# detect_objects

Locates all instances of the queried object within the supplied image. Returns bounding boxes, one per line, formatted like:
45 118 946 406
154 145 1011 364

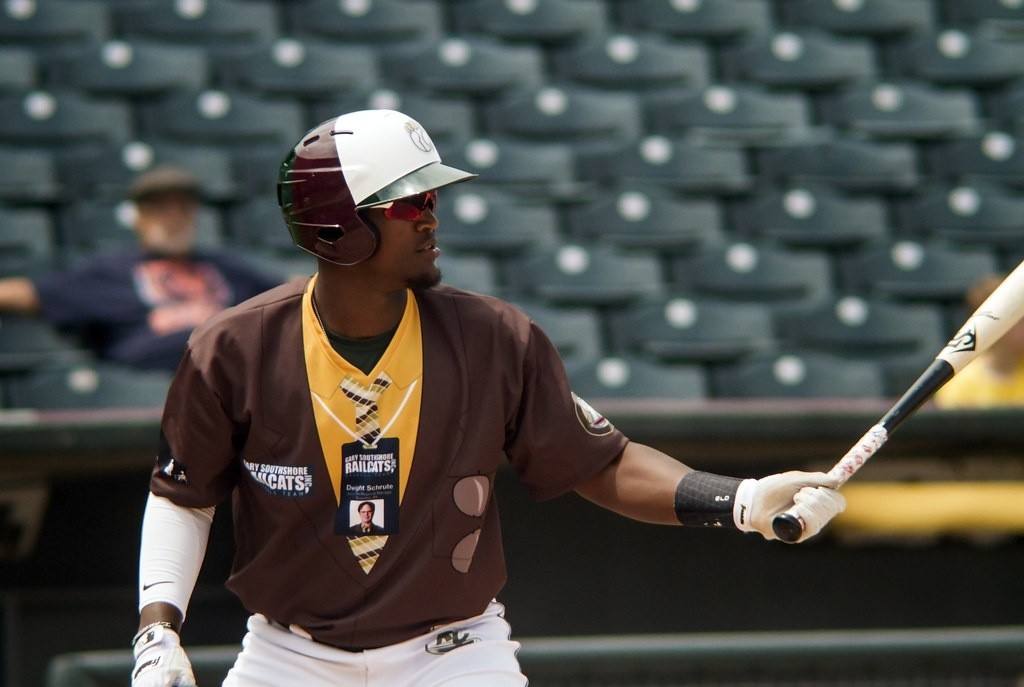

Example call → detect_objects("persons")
935 275 1024 410
131 108 847 687
0 165 285 376
351 502 382 536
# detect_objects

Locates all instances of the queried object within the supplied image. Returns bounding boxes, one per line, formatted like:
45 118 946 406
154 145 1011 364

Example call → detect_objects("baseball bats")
772 256 1024 545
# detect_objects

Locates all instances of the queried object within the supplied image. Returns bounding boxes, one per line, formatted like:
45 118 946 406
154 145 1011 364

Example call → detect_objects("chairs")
0 0 1024 409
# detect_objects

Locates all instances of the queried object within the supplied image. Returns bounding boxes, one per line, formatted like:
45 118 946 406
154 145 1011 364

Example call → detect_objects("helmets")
276 106 479 270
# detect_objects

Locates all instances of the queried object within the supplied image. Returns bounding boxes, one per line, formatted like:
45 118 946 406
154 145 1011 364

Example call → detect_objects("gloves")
130 626 195 687
733 469 848 545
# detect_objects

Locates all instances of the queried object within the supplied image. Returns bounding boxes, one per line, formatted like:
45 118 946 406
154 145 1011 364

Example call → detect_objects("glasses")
358 186 438 224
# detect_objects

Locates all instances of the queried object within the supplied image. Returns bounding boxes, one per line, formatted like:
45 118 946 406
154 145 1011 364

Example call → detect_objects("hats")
130 165 205 202
